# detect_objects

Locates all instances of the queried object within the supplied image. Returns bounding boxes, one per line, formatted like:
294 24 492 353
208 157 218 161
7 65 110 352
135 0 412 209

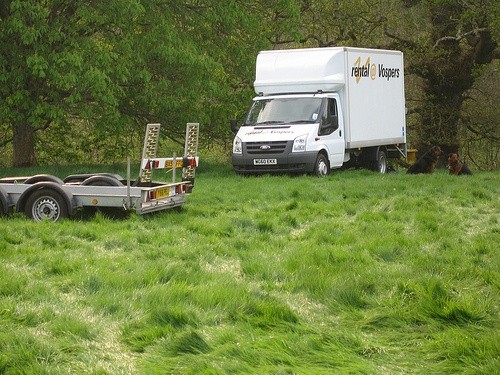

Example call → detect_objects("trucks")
230 47 407 177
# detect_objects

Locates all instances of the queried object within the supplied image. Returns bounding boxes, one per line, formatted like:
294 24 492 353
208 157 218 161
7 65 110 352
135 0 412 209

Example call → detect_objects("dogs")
405 146 444 174
446 153 472 175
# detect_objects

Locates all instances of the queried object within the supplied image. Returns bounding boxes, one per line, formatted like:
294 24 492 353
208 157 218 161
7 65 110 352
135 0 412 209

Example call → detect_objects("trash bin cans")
404 148 417 168
